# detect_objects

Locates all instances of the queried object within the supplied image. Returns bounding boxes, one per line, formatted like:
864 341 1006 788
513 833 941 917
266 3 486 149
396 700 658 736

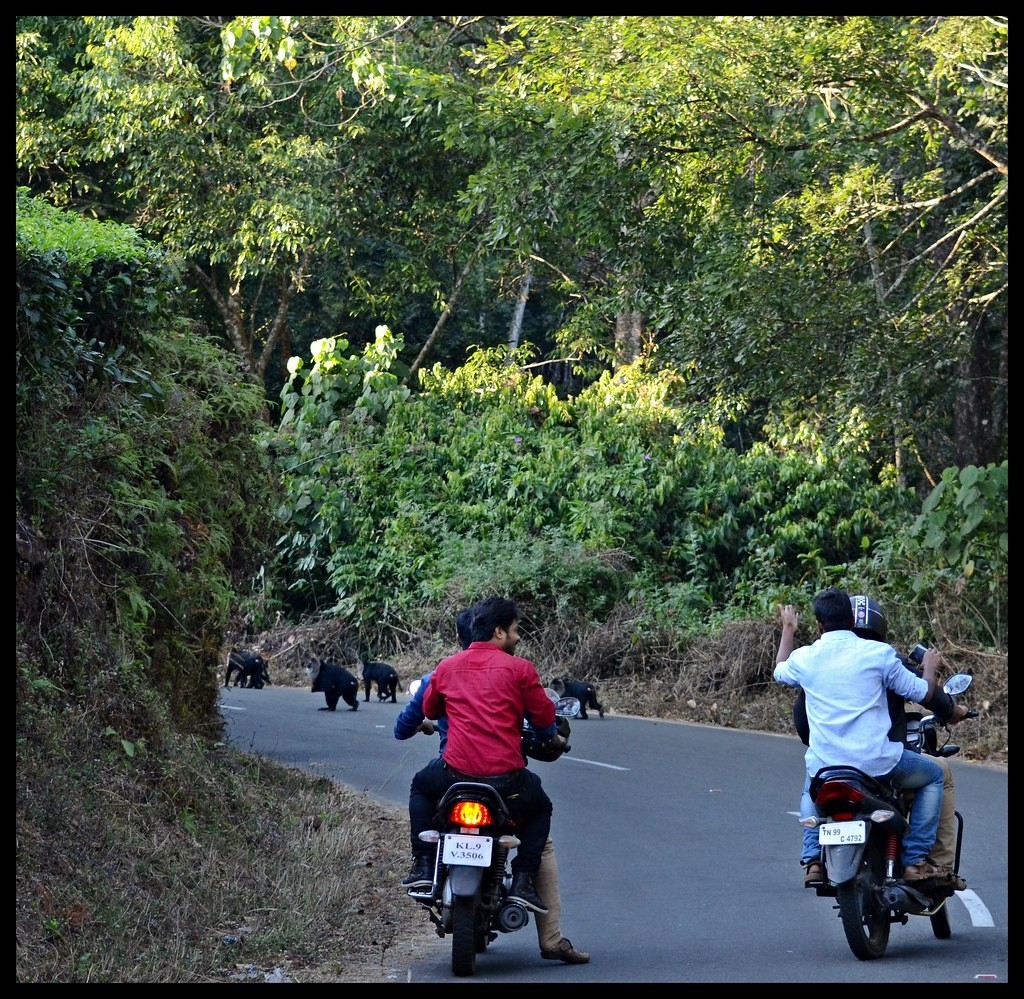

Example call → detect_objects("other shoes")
539 937 590 964
939 869 967 891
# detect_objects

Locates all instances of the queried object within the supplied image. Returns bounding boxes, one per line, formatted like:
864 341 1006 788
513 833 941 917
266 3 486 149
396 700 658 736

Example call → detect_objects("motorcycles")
408 679 584 975
807 672 981 963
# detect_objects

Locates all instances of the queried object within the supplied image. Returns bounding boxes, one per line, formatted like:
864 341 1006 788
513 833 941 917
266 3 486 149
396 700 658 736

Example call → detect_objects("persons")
401 597 556 914
773 588 949 883
794 595 969 890
393 609 590 964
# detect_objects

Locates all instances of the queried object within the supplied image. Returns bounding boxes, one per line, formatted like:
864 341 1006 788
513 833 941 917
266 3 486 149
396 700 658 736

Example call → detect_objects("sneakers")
400 855 434 887
505 870 549 914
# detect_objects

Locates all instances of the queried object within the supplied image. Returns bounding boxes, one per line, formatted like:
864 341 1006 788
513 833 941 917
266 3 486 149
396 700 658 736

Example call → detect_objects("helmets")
848 594 888 642
521 716 571 762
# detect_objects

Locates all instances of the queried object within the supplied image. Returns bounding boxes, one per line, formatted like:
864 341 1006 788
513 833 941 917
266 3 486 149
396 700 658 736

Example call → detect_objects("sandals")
903 860 944 880
800 860 824 888
